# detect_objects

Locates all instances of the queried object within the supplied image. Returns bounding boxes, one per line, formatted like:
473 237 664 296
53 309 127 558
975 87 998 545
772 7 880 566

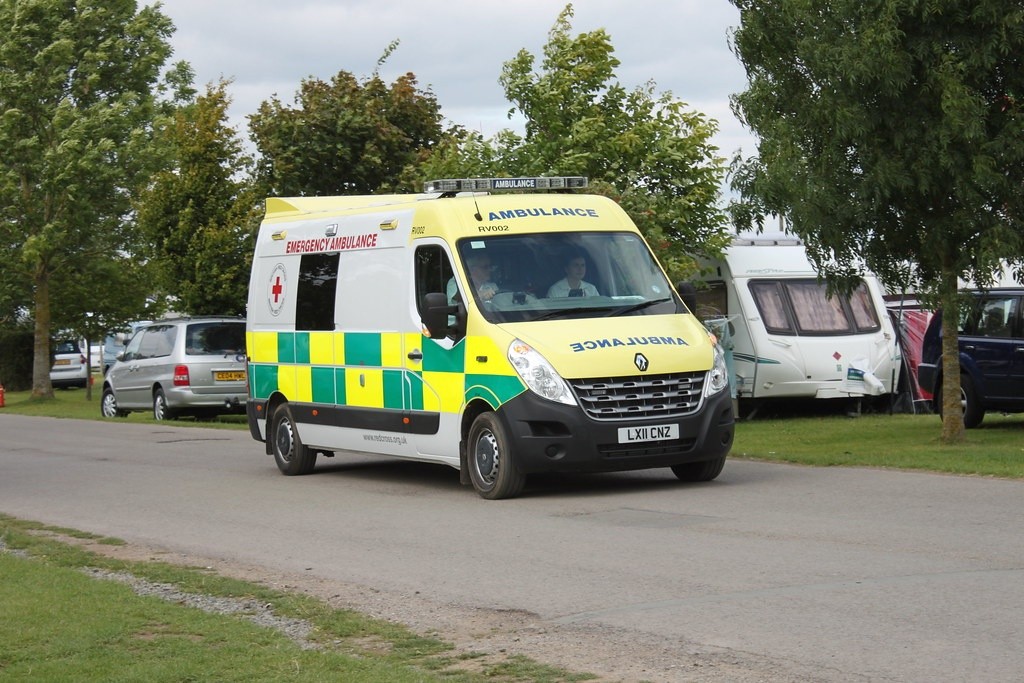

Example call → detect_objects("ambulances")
244 175 736 501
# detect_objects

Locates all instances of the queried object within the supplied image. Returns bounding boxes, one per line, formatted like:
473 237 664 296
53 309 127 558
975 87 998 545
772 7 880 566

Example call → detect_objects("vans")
46 335 88 390
102 319 155 376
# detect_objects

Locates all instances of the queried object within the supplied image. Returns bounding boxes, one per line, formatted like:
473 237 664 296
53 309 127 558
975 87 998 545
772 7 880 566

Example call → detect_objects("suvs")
918 286 1024 430
101 313 248 423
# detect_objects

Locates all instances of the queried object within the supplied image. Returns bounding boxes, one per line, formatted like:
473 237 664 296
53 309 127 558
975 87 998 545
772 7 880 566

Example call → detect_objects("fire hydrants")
0 384 6 408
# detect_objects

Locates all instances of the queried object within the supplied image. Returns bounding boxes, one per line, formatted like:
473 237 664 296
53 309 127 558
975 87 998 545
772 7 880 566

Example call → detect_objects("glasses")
470 262 498 272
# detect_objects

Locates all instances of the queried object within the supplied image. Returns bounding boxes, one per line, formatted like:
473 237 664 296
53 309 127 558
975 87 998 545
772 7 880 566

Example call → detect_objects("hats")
557 251 589 268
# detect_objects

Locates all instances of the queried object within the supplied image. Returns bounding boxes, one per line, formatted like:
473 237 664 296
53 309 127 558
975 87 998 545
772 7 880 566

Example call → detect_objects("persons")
546 251 600 298
447 252 499 326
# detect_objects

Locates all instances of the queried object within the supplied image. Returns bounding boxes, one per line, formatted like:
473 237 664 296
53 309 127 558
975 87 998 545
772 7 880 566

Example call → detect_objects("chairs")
1005 312 1024 338
533 254 602 299
977 308 1008 337
